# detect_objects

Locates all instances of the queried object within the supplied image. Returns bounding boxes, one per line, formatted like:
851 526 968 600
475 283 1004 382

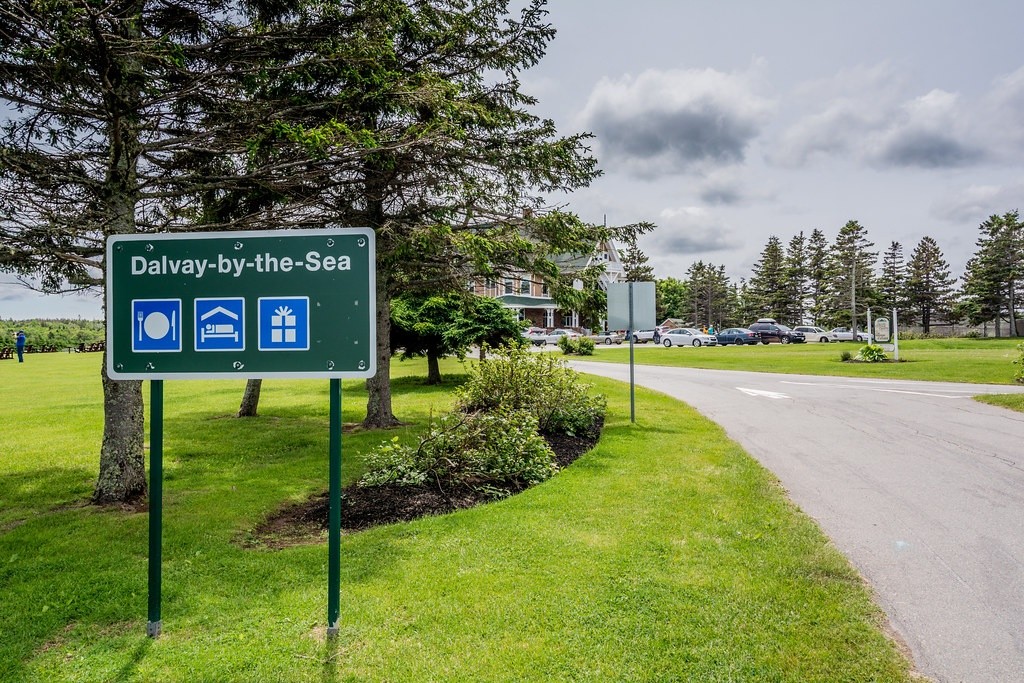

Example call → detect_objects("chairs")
0 342 105 359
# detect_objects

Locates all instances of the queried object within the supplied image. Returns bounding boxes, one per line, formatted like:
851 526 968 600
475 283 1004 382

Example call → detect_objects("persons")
14 330 25 363
653 328 662 344
702 322 721 336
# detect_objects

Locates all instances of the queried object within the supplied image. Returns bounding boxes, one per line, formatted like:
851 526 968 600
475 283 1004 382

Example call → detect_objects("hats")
19 331 23 333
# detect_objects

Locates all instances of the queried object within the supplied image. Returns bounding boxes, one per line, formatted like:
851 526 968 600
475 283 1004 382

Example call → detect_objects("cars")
587 330 625 345
829 326 875 343
519 326 546 348
793 325 839 342
713 327 762 346
660 327 718 347
542 328 583 346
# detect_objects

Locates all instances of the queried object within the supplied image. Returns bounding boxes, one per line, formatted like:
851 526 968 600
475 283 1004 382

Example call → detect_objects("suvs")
632 325 671 344
747 317 806 345
